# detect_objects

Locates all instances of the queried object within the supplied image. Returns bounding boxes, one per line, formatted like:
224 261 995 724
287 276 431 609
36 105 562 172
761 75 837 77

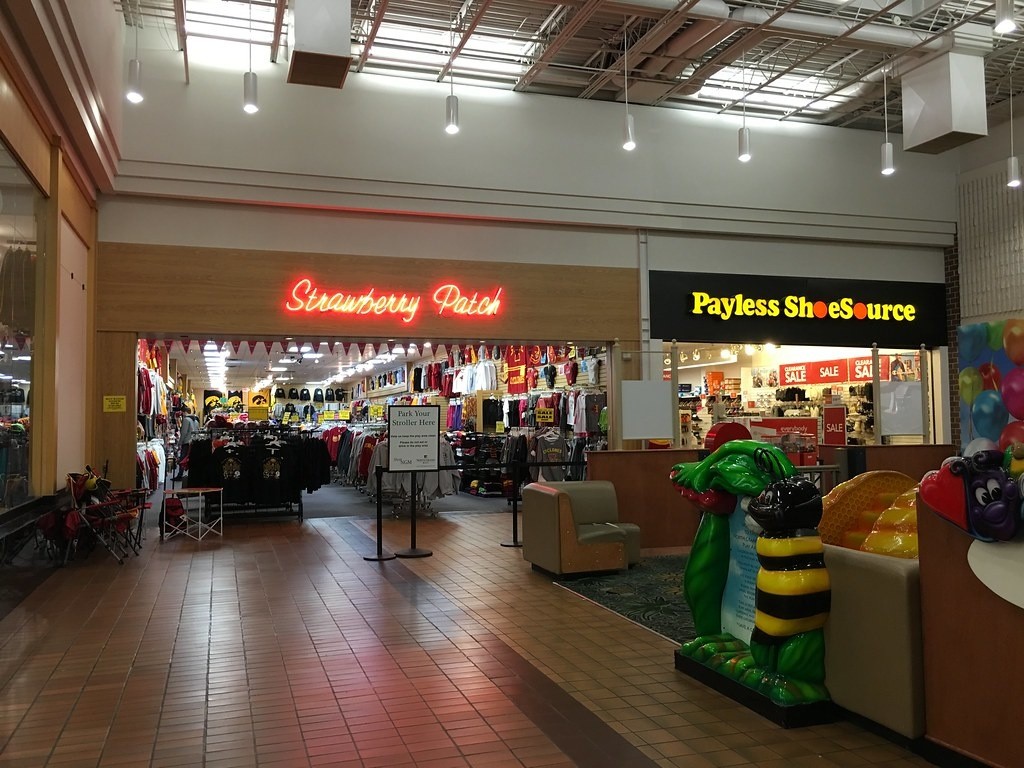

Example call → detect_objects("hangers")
478 358 491 364
488 383 604 395
192 425 312 451
137 442 155 452
347 424 380 437
139 360 157 377
507 426 559 441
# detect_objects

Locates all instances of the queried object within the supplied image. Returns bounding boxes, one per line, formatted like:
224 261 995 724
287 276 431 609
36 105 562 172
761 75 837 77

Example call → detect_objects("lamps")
297 354 304 364
995 0 1015 33
880 50 894 175
622 15 636 150
738 46 752 162
126 0 142 104
1007 63 1021 187
290 355 295 361
243 0 258 114
445 0 459 135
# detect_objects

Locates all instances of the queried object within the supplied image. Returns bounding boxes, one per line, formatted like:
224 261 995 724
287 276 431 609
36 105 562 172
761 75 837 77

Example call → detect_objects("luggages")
158 457 187 536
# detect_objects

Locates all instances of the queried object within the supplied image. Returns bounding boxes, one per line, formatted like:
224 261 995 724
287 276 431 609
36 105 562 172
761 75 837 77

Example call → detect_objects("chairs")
524 480 643 576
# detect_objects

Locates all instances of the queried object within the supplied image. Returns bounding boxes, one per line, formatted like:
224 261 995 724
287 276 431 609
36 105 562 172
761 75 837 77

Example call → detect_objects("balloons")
959 319 1024 456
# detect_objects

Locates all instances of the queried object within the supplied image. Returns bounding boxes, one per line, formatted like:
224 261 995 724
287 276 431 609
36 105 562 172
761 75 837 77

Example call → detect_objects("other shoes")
170 475 182 481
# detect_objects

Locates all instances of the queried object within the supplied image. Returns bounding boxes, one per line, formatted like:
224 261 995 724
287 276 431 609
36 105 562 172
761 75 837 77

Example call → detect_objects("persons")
170 408 195 481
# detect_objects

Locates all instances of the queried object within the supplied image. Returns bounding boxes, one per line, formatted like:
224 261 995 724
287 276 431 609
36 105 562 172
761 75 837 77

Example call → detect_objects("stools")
162 487 223 541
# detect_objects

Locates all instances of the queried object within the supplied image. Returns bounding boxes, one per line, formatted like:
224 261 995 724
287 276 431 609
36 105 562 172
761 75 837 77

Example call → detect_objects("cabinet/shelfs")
444 433 506 495
678 394 741 447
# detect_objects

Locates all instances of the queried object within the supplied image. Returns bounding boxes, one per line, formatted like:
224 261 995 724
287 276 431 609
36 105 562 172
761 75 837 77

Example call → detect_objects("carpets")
554 553 696 648
